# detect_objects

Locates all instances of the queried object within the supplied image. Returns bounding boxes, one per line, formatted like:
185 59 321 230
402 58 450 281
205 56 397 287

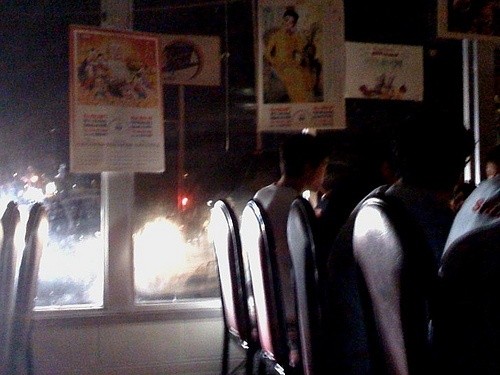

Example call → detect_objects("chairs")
14 200 50 375
428 220 500 375
206 198 265 374
239 199 304 375
0 200 22 375
337 195 433 375
286 195 359 375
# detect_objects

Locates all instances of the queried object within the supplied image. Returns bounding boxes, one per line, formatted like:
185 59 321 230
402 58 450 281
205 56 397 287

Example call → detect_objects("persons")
233 128 500 375
265 8 317 103
